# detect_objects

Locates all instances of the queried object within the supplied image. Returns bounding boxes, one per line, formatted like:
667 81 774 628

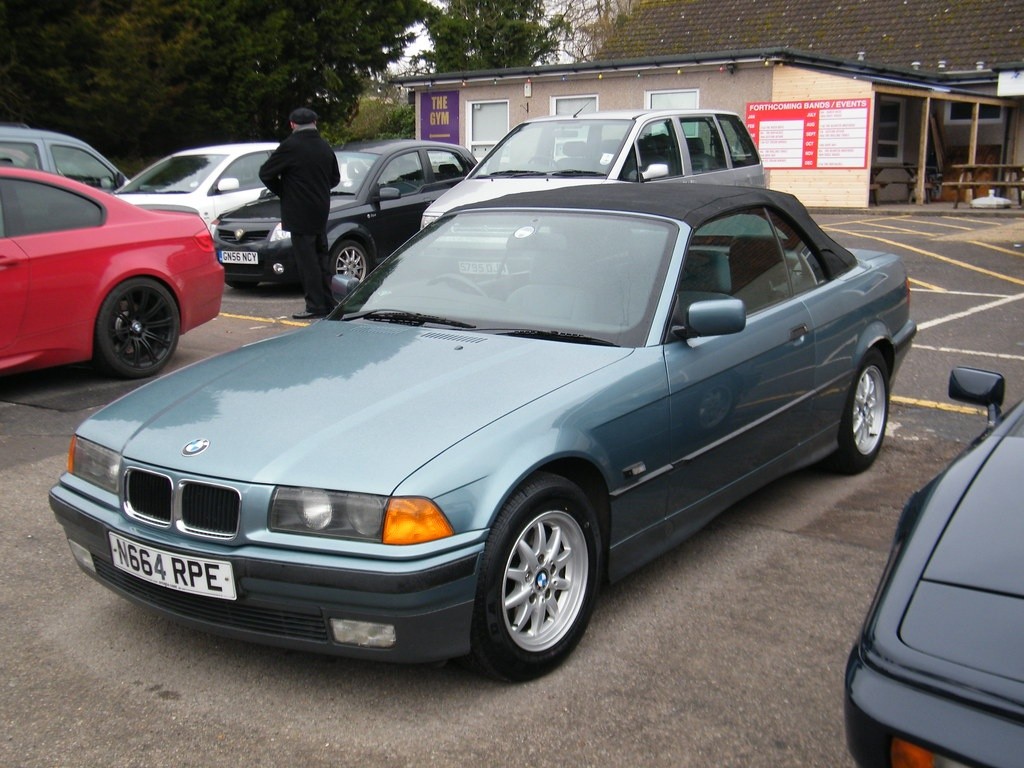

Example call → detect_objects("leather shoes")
292 310 329 319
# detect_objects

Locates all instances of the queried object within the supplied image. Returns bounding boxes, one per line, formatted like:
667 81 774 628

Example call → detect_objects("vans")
413 108 769 273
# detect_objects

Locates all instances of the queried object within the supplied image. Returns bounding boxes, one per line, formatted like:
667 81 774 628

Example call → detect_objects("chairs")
555 141 595 172
347 161 367 190
627 139 671 181
399 161 420 184
729 234 782 286
602 138 621 153
438 164 460 176
682 249 732 296
685 136 715 172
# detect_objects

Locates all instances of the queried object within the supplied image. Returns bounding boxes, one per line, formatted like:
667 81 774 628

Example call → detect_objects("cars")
108 137 280 248
209 136 481 300
44 180 917 675
0 168 226 381
841 365 1023 767
0 122 132 200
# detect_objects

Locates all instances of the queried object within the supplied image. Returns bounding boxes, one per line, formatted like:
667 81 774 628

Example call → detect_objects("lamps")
911 62 921 70
856 51 865 61
976 62 984 70
938 61 946 71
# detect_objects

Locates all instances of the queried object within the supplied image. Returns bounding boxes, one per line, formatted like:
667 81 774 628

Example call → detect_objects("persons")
259 108 341 320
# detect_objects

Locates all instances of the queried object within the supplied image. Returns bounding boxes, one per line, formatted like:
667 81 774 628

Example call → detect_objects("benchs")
941 182 1024 188
875 179 935 184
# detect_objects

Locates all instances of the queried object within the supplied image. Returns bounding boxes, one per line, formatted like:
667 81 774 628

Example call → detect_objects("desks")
952 164 1024 209
873 163 938 204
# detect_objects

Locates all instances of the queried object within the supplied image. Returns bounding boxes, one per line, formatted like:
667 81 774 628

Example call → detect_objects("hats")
289 108 318 125
